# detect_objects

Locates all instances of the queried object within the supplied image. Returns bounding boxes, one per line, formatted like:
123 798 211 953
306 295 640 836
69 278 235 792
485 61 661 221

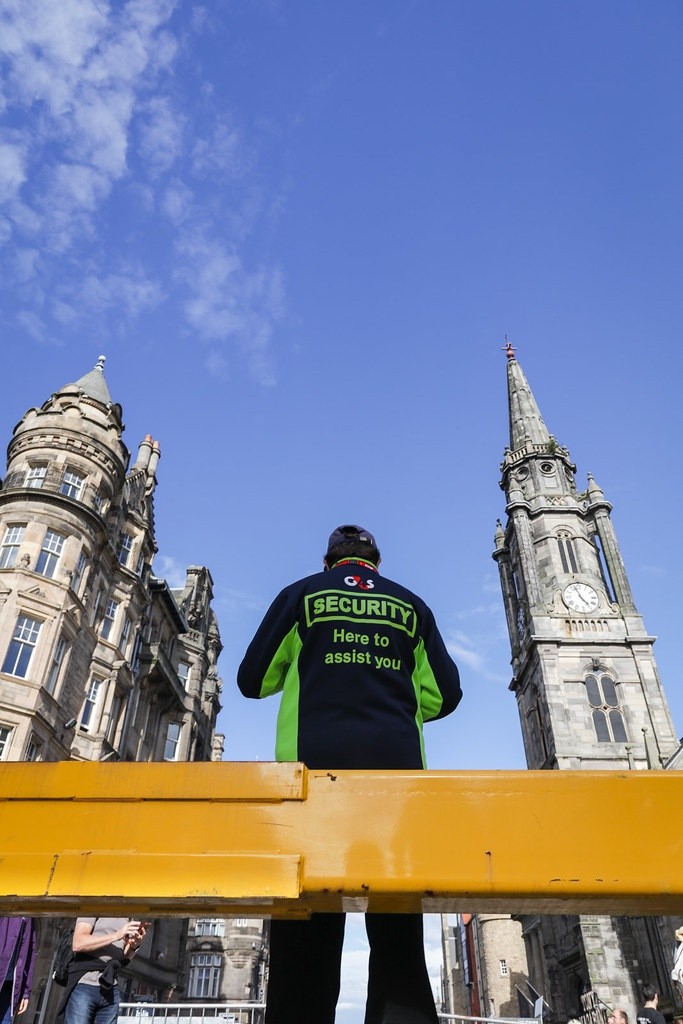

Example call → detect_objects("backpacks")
52 918 99 987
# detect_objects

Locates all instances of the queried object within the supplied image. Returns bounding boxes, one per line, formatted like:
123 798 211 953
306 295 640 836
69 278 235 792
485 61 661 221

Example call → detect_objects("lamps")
65 718 78 729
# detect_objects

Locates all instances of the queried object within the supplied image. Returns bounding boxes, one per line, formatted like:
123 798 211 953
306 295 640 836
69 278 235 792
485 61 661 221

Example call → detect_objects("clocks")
561 581 601 614
514 603 526 640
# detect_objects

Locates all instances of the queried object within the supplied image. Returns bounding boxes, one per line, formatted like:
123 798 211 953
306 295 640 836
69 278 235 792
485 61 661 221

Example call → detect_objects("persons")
63 916 149 1024
568 986 683 1024
236 523 464 1024
0 916 37 1024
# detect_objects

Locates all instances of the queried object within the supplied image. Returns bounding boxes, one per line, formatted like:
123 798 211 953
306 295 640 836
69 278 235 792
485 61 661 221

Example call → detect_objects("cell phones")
137 922 153 935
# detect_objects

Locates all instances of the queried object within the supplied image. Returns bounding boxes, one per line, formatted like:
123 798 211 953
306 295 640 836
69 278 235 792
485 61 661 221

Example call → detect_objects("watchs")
130 945 141 952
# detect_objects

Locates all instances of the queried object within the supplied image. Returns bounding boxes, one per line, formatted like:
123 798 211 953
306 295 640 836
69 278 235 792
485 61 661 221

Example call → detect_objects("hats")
328 524 378 550
